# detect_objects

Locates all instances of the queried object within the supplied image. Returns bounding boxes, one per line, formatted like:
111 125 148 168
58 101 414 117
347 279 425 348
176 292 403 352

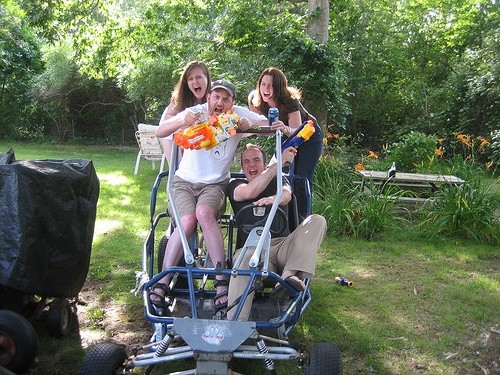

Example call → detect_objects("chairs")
134 123 168 175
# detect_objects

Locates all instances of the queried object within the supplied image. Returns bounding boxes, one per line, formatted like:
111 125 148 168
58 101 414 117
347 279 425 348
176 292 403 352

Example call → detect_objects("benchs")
354 181 440 188
381 196 428 205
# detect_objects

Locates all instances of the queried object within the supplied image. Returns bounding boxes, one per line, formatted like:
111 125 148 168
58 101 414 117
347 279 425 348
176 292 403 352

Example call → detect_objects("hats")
210 79 236 98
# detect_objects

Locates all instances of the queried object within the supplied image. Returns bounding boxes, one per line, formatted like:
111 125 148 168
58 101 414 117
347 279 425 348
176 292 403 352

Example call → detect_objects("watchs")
287 126 291 134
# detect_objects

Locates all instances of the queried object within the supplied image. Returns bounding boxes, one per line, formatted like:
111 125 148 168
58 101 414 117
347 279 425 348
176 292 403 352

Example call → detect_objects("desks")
358 170 468 194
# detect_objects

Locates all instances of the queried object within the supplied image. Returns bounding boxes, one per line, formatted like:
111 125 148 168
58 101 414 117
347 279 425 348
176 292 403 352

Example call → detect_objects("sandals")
149 282 170 304
213 279 228 309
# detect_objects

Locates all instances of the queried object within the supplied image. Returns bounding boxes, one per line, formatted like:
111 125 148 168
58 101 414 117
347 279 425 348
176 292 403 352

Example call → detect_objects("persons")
226 144 327 321
159 61 212 170
149 80 269 309
252 67 323 233
248 89 259 114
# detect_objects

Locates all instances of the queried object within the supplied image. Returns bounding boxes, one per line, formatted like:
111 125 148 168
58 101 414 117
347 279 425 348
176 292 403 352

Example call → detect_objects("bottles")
334 276 354 288
388 161 396 178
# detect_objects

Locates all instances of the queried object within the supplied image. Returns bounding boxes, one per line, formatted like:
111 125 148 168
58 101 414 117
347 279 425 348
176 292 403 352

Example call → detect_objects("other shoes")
272 275 304 302
